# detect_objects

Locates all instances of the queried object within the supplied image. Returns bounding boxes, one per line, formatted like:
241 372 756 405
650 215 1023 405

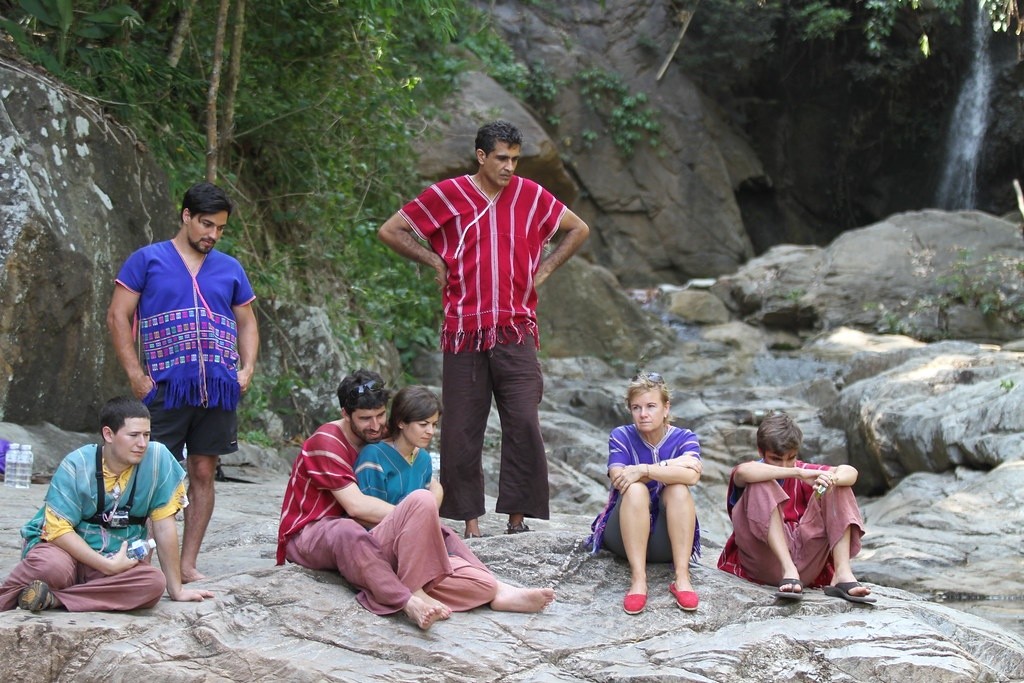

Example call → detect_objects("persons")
716 413 878 604
376 119 590 540
276 367 443 634
584 370 704 620
353 384 555 621
0 395 215 624
106 180 262 581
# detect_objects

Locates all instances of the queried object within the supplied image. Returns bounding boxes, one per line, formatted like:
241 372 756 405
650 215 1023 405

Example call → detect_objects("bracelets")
645 463 651 479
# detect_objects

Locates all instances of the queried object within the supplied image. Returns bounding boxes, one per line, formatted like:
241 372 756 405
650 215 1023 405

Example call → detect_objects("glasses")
343 379 388 406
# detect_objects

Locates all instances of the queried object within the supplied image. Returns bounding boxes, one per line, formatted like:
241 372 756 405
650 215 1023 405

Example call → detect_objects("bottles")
4 443 33 488
104 538 156 560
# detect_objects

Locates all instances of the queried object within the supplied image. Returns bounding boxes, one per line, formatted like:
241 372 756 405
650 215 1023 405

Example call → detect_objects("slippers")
824 579 876 602
776 579 803 599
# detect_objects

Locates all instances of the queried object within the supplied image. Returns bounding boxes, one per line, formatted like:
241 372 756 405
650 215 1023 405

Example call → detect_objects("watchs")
658 459 668 467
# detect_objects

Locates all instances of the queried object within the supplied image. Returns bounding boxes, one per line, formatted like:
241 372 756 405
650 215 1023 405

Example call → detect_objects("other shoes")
18 581 54 612
507 519 536 534
669 580 700 610
624 585 648 614
